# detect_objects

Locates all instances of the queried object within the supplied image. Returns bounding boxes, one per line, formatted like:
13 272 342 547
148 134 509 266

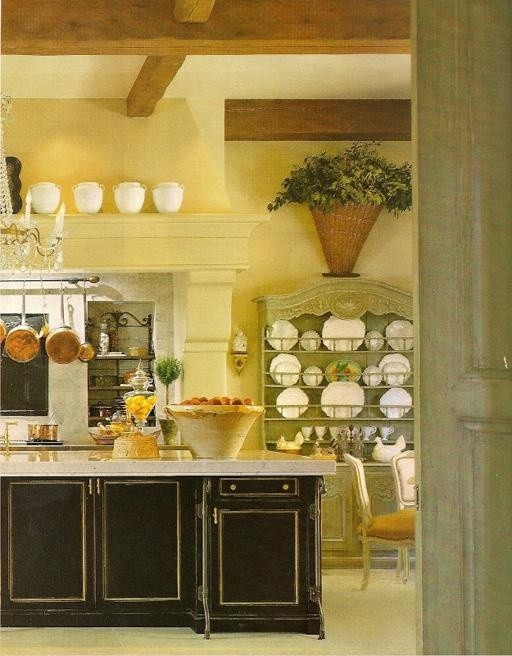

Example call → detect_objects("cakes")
111 435 161 459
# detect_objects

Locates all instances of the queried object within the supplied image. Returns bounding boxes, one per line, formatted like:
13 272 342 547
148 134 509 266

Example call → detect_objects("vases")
27 181 64 213
73 181 105 213
151 182 185 212
112 182 148 213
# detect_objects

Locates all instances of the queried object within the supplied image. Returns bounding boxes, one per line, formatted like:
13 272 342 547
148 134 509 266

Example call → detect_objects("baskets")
311 202 384 274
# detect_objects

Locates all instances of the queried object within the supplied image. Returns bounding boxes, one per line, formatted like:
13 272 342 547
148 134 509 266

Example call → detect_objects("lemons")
126 395 157 421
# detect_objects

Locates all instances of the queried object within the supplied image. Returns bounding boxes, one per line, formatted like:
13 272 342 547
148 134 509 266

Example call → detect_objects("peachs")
179 396 253 405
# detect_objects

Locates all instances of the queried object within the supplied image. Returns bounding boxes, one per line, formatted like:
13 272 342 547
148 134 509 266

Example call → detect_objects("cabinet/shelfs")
191 477 330 640
85 306 156 428
253 274 415 569
1 477 190 631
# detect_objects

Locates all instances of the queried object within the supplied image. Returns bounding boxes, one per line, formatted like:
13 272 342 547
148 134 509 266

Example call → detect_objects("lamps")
1 90 67 282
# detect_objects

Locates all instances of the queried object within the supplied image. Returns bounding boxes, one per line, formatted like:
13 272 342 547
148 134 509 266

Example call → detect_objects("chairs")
345 454 415 594
393 450 416 587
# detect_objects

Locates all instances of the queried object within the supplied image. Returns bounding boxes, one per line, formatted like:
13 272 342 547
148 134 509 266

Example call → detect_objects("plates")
320 380 365 418
276 387 311 421
266 316 415 386
378 389 413 418
278 448 302 455
113 397 126 415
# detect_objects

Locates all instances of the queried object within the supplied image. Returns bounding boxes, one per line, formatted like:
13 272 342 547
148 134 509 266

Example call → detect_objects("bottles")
336 430 363 459
96 319 110 355
124 359 160 434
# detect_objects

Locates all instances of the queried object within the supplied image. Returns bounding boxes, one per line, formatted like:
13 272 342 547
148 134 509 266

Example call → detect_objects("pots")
5 281 41 363
45 281 95 365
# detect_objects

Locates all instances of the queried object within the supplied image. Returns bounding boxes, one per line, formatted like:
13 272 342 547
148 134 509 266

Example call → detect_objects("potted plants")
265 138 413 280
152 353 183 445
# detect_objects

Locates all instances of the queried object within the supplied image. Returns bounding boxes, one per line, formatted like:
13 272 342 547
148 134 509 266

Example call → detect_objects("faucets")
3 421 20 448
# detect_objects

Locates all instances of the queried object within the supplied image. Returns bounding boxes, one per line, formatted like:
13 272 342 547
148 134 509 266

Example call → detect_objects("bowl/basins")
129 348 145 357
91 400 112 418
94 375 123 387
26 423 59 441
162 405 265 461
95 437 114 445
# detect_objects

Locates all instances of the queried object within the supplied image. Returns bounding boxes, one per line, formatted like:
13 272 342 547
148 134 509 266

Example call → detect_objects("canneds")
27 424 59 441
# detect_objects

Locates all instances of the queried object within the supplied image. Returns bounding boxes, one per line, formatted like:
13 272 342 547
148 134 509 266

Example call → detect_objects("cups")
299 425 395 442
